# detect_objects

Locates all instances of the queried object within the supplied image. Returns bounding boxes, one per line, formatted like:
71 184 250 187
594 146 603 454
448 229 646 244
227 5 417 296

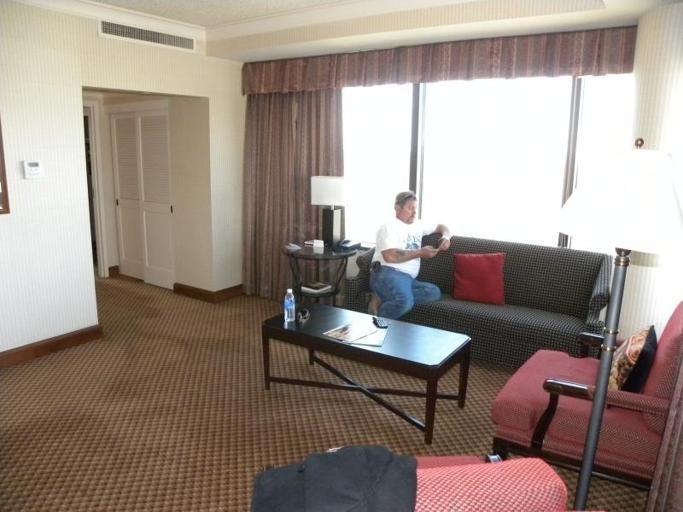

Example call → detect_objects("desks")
281 244 358 308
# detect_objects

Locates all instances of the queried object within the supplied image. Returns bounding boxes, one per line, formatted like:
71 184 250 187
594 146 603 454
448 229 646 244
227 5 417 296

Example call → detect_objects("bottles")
283 288 296 322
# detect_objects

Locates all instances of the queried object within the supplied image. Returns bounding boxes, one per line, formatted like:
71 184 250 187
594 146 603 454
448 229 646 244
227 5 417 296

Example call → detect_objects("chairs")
249 443 569 511
485 300 682 499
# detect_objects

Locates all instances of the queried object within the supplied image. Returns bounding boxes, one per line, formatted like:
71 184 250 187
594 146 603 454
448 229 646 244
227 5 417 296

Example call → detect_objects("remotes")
372 316 388 328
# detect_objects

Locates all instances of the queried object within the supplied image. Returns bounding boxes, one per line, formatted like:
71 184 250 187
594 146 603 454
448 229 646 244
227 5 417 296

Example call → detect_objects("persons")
368 190 453 321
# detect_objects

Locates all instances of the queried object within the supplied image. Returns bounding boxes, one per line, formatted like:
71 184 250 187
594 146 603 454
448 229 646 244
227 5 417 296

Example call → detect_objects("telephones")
337 239 361 250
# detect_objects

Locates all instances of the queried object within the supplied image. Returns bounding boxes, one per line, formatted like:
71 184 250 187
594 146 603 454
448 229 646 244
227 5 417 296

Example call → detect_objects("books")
300 281 333 294
322 321 388 348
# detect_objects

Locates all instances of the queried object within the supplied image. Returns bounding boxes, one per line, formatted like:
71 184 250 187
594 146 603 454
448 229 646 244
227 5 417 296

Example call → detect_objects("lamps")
311 175 345 244
554 143 681 511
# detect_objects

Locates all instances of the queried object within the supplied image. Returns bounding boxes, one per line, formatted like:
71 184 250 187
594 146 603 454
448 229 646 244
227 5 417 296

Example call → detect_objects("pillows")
450 250 506 306
608 325 655 391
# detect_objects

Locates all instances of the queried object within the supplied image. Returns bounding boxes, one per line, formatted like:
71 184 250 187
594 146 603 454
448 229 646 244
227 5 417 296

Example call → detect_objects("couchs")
350 237 613 370
